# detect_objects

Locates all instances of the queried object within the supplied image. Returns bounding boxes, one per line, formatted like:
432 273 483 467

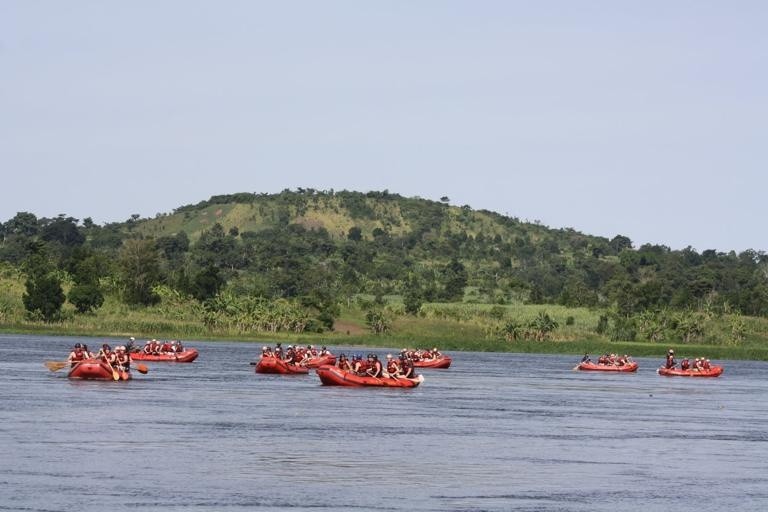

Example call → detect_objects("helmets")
130 337 181 345
262 341 326 355
669 349 710 363
340 347 438 360
74 343 127 352
585 352 628 362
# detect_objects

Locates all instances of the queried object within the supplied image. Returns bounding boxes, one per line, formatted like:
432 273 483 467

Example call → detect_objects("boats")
256 357 451 387
68 342 197 380
577 362 723 376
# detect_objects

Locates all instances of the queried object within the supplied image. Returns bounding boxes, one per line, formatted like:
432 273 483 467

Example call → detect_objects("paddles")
44 361 81 372
174 351 179 361
114 351 130 381
249 361 258 366
573 359 590 371
101 350 120 381
404 378 420 383
130 362 148 374
384 370 405 387
367 372 391 387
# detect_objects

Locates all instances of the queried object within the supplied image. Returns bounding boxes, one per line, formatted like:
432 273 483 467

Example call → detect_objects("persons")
400 347 440 361
144 338 184 354
665 347 714 372
68 343 131 371
261 342 330 366
580 350 637 368
338 352 414 378
127 337 137 352
